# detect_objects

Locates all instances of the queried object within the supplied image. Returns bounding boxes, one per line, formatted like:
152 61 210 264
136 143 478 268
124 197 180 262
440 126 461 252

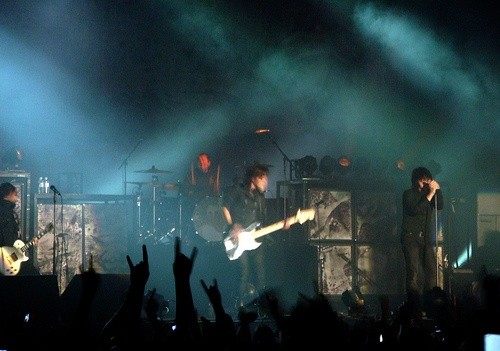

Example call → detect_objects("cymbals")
143 169 173 173
155 183 199 191
127 182 147 185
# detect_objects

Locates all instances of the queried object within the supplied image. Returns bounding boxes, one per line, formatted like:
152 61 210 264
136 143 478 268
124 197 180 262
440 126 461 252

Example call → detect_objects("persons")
0 182 39 288
400 167 444 303
0 236 500 351
221 165 290 312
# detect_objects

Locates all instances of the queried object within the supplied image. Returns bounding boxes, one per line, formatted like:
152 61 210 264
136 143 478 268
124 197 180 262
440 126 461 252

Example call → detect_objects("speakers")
32 194 135 295
303 188 405 299
53 273 132 319
0 275 60 314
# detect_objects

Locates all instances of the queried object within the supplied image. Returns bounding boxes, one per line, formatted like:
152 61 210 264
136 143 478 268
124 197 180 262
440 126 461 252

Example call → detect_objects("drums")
190 193 231 242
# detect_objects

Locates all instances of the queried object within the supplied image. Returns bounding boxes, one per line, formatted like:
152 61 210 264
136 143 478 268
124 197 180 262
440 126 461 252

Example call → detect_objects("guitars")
223 207 317 260
0 223 56 275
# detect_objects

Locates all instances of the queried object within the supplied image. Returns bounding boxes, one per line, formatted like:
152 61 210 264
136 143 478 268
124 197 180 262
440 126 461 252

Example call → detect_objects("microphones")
50 185 62 198
255 128 272 135
139 136 147 139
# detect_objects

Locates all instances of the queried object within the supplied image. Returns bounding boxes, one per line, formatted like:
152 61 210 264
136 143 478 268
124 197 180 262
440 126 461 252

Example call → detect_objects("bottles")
38 177 50 194
